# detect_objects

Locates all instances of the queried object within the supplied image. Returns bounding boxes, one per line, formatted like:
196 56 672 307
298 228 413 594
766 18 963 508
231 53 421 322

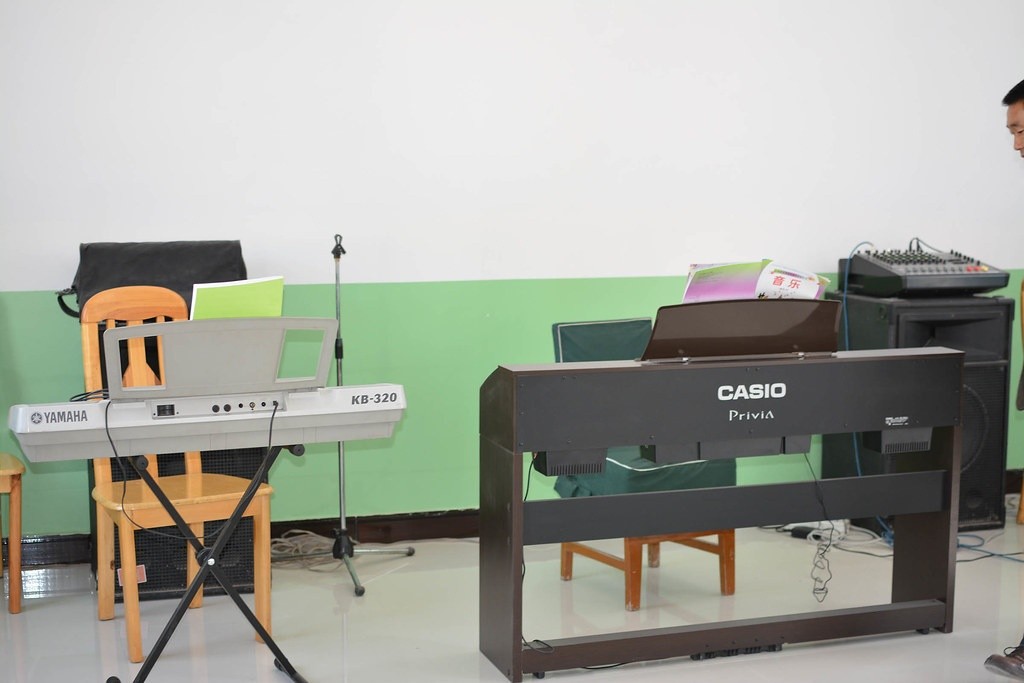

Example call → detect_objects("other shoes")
984 643 1024 683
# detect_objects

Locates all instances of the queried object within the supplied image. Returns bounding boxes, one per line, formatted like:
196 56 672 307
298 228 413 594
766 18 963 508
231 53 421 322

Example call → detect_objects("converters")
792 527 821 540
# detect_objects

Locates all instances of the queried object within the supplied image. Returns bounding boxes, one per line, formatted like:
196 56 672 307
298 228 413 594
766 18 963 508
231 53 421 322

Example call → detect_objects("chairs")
0 451 26 615
81 285 273 663
553 317 736 611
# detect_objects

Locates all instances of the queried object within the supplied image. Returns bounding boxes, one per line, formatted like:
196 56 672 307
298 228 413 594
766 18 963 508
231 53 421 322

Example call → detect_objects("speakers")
822 291 1015 538
88 447 270 603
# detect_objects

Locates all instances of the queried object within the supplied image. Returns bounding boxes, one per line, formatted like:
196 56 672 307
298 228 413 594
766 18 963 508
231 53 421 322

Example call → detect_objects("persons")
983 79 1024 683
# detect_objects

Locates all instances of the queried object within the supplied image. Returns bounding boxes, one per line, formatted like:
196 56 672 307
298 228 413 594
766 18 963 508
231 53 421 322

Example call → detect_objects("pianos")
9 317 406 683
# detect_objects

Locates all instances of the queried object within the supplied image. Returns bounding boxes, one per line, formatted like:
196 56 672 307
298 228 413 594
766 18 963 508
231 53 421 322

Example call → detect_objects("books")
189 275 283 321
681 254 833 305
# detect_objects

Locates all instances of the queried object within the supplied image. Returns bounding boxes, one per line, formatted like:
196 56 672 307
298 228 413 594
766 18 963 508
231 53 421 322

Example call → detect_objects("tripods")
271 235 416 596
